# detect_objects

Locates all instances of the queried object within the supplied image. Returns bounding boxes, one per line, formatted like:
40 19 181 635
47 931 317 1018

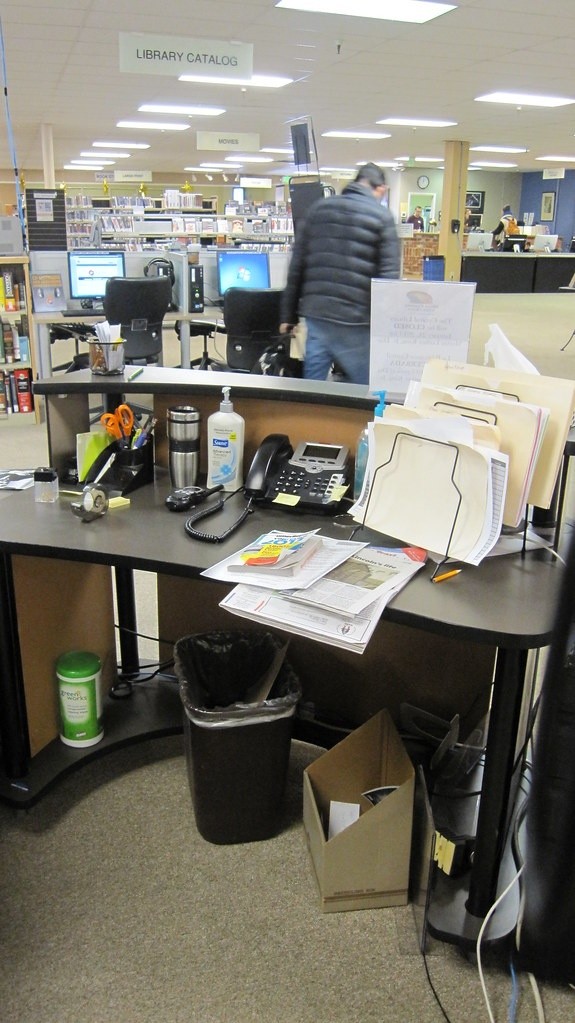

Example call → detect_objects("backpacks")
504 217 520 236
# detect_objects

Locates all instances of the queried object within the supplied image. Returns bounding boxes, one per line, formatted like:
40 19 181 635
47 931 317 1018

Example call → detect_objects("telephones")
244 434 353 515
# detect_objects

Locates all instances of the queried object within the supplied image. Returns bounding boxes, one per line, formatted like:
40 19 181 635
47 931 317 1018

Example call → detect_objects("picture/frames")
541 192 556 221
465 192 482 208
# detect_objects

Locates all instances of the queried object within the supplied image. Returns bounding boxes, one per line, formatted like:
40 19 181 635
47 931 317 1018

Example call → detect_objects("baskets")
87 338 126 376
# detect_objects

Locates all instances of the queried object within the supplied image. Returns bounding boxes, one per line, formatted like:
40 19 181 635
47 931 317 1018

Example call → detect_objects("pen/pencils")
131 418 159 449
128 368 143 380
433 570 462 583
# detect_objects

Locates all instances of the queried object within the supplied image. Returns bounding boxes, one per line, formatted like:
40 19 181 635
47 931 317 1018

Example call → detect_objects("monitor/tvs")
467 233 493 253
503 234 527 253
68 251 126 308
533 235 558 253
217 251 271 296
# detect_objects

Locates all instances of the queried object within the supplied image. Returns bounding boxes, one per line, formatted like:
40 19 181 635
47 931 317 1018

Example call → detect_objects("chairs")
175 287 296 374
48 275 173 424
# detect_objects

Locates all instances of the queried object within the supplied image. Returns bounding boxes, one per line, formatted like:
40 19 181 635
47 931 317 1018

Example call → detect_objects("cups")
56 650 105 749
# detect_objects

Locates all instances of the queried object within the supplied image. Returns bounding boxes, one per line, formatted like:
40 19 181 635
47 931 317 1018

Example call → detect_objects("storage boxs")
302 708 415 913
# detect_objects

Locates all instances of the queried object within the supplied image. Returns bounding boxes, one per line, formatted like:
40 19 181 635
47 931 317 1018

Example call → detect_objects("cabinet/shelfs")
0 253 46 424
64 195 296 239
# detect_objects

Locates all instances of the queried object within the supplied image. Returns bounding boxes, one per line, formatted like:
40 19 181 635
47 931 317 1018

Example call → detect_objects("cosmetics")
205 387 245 492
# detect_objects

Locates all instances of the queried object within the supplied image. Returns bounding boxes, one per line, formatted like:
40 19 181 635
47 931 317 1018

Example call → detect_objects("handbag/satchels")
248 333 305 379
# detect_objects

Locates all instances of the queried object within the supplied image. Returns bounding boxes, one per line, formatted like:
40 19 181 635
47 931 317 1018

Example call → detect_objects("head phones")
144 257 175 286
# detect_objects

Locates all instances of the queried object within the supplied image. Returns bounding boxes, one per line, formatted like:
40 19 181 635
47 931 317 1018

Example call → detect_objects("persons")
492 206 517 248
279 161 401 386
406 207 424 231
464 209 473 233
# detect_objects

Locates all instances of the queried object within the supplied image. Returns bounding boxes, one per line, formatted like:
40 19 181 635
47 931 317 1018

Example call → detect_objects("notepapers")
108 496 130 508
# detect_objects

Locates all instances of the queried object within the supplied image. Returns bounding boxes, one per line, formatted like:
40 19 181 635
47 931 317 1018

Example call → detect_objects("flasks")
166 405 201 489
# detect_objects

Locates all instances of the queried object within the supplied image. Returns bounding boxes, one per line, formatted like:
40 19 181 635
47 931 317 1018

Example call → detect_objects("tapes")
88 490 106 512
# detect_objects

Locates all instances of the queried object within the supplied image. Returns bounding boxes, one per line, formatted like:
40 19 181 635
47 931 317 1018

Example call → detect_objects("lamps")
191 172 240 182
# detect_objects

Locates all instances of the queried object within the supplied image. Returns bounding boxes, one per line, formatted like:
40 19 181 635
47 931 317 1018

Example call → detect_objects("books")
0 272 32 414
67 190 295 253
227 528 322 576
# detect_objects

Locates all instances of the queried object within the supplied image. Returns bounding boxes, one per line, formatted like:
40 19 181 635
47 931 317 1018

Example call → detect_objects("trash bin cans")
423 256 445 280
175 631 302 844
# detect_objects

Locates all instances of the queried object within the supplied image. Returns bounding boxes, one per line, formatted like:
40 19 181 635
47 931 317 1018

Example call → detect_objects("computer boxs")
188 263 204 313
147 261 173 312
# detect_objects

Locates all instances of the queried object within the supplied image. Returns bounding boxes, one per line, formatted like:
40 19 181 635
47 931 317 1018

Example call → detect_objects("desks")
1 364 574 950
461 251 575 292
30 305 226 369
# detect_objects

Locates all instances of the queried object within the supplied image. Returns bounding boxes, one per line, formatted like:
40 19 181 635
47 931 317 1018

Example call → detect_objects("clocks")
417 176 429 189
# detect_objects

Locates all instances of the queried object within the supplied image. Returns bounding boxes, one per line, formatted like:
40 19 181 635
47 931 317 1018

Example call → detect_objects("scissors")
101 404 134 449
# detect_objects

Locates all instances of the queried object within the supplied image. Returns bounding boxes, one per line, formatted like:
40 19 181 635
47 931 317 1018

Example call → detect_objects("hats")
503 204 510 212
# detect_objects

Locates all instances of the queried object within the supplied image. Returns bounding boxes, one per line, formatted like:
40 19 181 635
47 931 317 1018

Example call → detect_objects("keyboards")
61 309 105 317
